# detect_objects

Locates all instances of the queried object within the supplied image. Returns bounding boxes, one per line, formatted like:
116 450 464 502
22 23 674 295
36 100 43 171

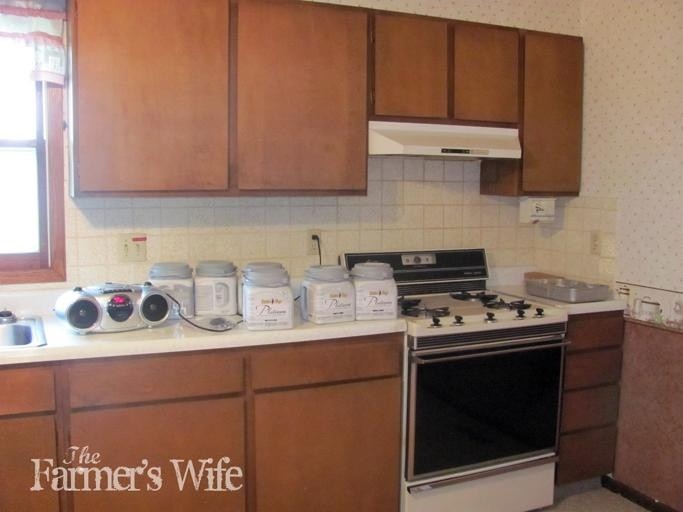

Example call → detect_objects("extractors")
367 118 522 160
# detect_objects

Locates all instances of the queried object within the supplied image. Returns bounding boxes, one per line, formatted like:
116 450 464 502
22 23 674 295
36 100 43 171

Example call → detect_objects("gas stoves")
399 289 564 328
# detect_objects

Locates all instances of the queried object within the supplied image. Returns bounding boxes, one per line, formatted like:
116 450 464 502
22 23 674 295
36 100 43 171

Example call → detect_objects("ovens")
406 335 571 482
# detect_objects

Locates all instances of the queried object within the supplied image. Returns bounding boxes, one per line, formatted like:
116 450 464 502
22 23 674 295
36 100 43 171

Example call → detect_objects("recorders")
54 281 169 334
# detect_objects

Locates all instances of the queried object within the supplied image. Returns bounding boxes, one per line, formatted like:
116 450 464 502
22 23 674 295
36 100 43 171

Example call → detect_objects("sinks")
0 314 47 351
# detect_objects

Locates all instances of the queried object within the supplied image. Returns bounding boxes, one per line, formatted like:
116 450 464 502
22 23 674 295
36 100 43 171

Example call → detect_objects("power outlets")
305 228 322 256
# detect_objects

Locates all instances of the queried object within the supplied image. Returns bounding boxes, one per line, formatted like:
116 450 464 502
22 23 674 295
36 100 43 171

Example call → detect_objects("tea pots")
617 285 640 316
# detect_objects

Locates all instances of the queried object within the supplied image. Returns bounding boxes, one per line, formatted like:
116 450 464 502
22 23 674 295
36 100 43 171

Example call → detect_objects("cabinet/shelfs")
480 31 584 196
554 311 625 504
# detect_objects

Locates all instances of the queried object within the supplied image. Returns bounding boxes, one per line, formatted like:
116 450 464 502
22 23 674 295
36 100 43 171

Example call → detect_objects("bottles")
641 296 663 324
148 260 399 331
